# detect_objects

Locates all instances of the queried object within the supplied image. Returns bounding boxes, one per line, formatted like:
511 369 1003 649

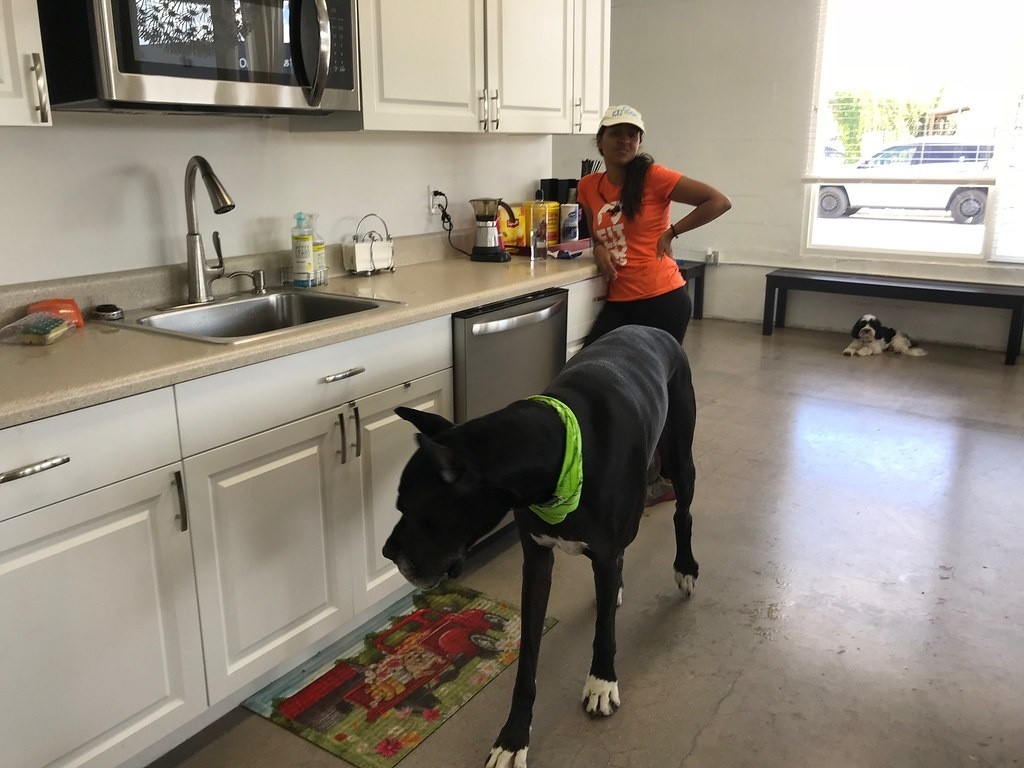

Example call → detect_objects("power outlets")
428 185 442 215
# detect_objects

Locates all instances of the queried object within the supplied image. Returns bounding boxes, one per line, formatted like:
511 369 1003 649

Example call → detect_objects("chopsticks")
581 159 602 178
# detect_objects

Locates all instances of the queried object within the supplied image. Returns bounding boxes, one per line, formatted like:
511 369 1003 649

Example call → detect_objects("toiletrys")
291 208 326 286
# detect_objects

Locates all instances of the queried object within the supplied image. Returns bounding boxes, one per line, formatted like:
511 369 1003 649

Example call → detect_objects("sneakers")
646 453 661 482
645 477 675 504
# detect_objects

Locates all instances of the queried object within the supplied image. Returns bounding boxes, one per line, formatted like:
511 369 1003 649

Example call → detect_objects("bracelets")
670 223 679 239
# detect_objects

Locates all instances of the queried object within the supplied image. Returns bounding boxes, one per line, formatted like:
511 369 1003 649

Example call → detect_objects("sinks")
90 285 410 346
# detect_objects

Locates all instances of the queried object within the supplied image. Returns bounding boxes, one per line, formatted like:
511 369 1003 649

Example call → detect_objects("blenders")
469 198 515 262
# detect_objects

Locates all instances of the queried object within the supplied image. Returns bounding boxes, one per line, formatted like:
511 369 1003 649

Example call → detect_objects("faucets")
184 155 236 304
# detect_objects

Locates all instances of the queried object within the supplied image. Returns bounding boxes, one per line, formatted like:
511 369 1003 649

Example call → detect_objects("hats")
598 104 646 136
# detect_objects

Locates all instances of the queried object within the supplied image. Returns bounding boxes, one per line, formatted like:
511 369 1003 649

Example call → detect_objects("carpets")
240 573 560 768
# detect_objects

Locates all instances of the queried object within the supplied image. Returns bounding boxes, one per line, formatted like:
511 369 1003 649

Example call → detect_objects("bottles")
292 213 325 286
531 190 547 261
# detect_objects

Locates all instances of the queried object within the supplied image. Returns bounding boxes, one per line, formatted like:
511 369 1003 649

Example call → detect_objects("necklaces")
597 171 623 215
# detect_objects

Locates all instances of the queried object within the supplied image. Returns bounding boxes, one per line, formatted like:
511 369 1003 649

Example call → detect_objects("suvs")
815 136 997 224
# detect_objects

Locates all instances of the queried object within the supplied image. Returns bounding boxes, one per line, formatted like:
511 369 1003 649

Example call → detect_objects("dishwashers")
452 288 568 424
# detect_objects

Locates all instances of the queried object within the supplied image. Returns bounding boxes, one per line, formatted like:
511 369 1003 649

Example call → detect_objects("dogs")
842 314 926 357
381 325 699 768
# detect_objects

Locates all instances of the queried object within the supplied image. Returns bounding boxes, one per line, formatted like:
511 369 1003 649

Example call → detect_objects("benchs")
677 260 706 319
762 268 1024 366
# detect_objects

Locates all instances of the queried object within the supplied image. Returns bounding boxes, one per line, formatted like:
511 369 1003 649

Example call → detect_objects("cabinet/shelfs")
289 0 573 135
0 385 208 768
560 275 608 363
0 0 53 126
572 0 611 135
173 314 454 719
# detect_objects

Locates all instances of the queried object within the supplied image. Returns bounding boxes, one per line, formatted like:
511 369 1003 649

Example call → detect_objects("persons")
576 104 732 508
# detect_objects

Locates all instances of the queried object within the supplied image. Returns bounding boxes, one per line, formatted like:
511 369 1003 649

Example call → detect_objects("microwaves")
37 0 362 119
